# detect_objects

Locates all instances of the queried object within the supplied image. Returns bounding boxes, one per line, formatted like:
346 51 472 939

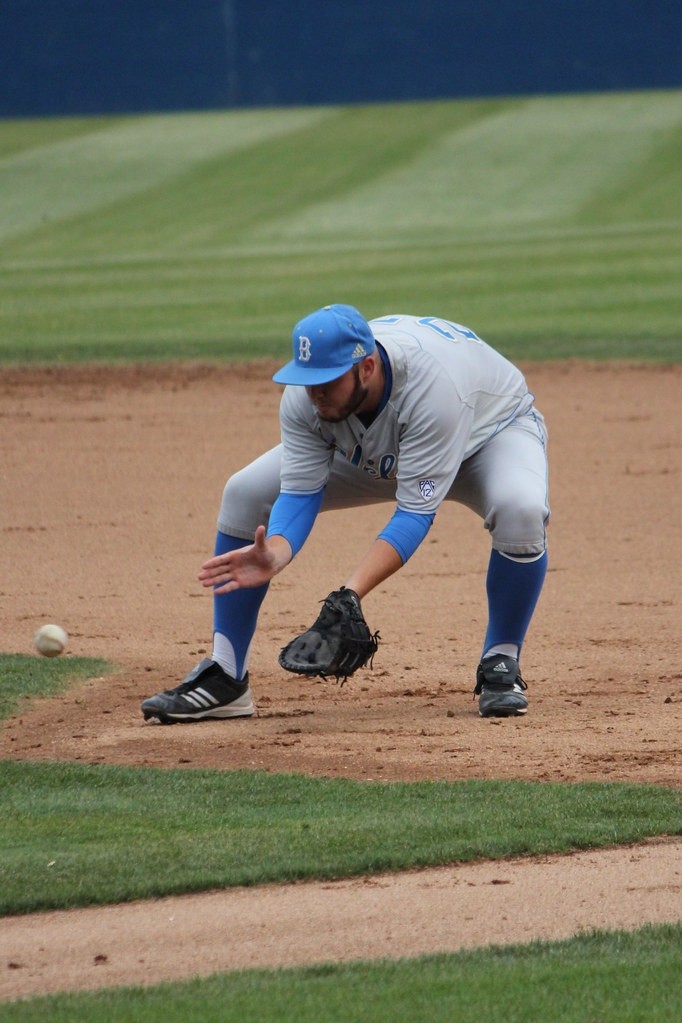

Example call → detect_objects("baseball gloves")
278 586 378 676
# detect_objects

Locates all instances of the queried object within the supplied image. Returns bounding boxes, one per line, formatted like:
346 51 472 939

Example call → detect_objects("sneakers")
141 658 254 723
472 653 529 717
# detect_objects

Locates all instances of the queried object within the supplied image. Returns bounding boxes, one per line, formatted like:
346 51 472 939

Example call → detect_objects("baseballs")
35 625 68 657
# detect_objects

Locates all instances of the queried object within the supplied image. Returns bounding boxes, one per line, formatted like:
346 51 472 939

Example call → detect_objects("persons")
139 303 553 722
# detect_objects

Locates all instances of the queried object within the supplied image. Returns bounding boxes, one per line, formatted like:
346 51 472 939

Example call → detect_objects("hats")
272 304 375 386
